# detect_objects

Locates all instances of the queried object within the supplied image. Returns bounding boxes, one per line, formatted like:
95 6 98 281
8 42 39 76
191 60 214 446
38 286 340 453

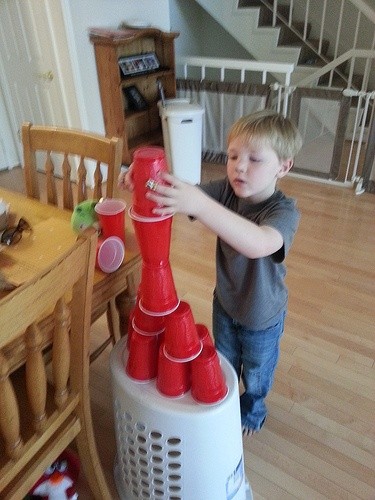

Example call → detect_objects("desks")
0 184 143 500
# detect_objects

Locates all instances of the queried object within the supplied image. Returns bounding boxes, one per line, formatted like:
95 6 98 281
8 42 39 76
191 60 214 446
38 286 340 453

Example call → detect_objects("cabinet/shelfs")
91 28 180 163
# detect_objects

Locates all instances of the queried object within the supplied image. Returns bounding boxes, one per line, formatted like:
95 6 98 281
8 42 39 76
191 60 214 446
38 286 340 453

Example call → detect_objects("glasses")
1 216 30 245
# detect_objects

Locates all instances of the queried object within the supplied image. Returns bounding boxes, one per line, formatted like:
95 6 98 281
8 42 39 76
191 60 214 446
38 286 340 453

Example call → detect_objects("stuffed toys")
28 447 82 500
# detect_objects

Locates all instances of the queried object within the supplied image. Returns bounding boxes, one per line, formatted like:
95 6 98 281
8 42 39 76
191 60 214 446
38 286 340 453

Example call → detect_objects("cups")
94 236 125 273
94 198 127 244
123 146 230 407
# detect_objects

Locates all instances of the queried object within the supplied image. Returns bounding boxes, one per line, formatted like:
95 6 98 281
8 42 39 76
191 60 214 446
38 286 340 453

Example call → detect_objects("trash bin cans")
158 97 205 186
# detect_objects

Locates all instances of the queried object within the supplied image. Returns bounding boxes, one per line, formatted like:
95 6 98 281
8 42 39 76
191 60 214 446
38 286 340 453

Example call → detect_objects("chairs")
0 225 112 500
20 120 124 217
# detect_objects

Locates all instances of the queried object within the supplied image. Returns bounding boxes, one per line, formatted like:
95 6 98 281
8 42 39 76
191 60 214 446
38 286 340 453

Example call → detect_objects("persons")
116 109 304 438
